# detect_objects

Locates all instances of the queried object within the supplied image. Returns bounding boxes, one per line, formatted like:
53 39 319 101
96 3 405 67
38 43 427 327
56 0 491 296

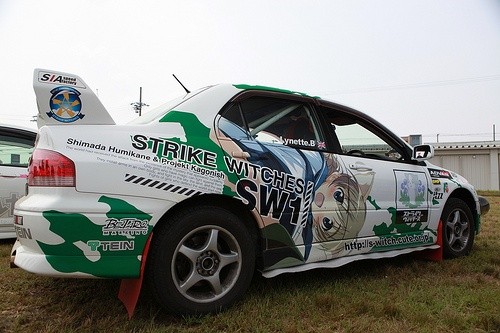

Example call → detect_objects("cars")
0 123 38 241
9 69 491 317
